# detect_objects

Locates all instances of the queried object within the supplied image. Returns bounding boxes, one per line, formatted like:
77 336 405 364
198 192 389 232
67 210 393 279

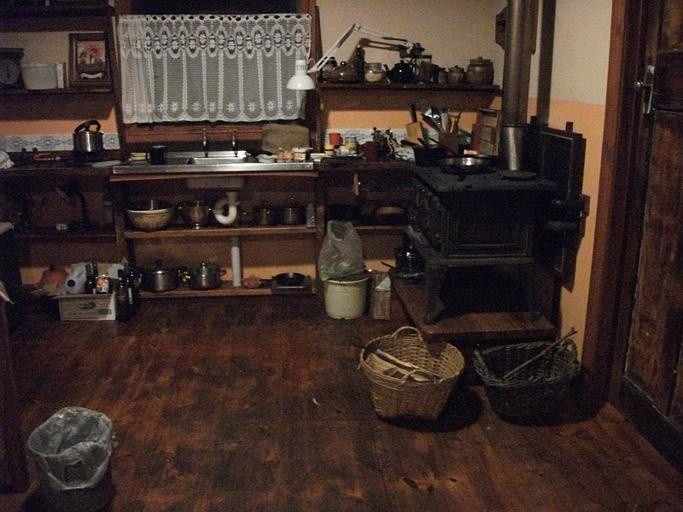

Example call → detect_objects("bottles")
116 264 140 323
420 56 494 85
320 45 383 82
85 259 98 294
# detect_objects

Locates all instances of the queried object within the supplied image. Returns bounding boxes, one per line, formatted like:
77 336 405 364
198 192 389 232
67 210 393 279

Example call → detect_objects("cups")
329 132 341 145
361 141 379 161
146 146 168 165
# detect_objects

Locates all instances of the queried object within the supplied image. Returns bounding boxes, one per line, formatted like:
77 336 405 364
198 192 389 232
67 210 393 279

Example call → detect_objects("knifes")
407 102 418 123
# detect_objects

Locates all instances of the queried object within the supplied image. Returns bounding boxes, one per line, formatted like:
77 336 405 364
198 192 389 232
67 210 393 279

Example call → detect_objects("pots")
418 138 489 174
253 196 305 225
400 140 445 167
139 259 226 292
393 243 424 275
259 273 305 285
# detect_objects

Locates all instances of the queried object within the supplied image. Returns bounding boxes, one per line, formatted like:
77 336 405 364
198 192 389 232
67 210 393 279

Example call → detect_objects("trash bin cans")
25 409 116 512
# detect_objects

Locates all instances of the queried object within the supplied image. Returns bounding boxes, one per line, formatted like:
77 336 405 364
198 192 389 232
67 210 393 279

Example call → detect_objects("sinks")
192 150 248 163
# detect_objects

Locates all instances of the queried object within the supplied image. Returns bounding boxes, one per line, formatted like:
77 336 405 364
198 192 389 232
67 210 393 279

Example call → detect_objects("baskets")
367 272 391 320
360 325 464 421
474 340 576 417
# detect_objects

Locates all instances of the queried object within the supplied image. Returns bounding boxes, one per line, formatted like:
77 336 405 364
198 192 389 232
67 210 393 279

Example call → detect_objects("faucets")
201 128 209 156
232 130 238 157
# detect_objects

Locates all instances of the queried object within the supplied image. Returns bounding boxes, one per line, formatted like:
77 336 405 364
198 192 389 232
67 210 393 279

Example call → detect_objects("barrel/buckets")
323 279 369 319
64 262 94 294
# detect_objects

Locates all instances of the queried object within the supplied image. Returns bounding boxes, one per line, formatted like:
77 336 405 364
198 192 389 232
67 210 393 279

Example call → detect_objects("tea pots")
383 60 420 83
72 120 105 155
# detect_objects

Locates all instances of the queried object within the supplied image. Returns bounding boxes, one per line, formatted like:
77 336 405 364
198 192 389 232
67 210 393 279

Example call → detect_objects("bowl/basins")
123 195 212 232
21 61 58 91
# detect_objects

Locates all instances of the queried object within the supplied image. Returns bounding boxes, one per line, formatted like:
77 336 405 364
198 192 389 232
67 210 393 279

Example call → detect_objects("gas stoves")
0 144 119 169
